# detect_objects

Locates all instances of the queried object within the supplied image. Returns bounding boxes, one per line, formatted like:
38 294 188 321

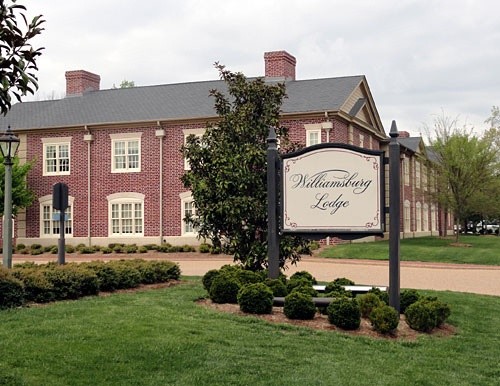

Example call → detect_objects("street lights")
0 122 21 267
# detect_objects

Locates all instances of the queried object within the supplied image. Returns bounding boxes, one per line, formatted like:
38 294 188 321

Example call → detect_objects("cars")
454 221 500 234
